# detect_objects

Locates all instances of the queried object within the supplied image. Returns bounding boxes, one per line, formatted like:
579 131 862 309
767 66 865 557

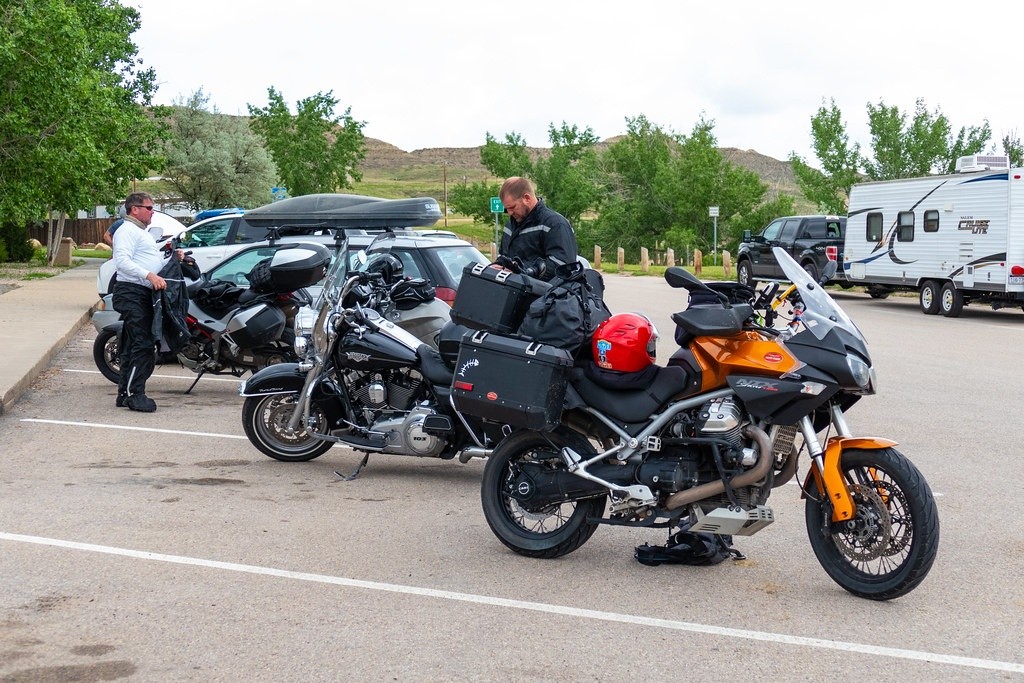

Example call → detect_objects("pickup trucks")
735 215 890 300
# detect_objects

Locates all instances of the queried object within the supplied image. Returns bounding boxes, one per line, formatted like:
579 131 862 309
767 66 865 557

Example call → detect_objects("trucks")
842 153 1024 319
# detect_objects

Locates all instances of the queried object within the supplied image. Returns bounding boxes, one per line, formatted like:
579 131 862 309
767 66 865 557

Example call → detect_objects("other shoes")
128 394 158 413
116 394 129 406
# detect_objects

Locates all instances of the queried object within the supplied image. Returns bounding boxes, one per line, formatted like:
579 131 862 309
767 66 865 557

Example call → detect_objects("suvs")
91 191 495 353
96 208 464 300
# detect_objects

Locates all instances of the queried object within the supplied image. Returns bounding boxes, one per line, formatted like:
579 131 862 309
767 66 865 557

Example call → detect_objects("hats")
120 203 127 218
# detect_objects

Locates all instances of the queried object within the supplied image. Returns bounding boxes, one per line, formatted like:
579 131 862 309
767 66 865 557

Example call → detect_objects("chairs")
827 227 836 236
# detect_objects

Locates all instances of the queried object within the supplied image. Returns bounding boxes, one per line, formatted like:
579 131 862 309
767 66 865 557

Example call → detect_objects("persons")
103 204 126 248
112 191 184 412
498 176 578 283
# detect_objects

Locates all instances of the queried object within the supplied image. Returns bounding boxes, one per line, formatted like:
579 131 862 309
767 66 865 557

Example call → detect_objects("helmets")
591 311 660 373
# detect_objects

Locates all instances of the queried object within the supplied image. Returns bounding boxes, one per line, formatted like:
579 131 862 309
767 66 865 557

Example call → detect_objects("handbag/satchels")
633 531 733 567
518 267 613 354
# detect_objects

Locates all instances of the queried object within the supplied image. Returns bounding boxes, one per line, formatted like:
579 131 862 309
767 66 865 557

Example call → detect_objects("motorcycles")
90 225 594 485
447 247 941 601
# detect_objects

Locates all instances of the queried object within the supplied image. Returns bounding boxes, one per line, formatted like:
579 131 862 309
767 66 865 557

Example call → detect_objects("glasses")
129 205 153 212
